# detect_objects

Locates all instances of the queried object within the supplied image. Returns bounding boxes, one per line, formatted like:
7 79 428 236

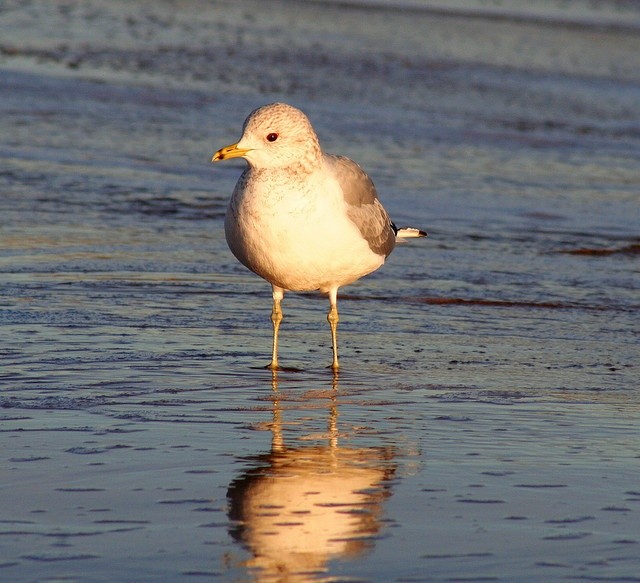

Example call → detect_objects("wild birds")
211 102 428 372
